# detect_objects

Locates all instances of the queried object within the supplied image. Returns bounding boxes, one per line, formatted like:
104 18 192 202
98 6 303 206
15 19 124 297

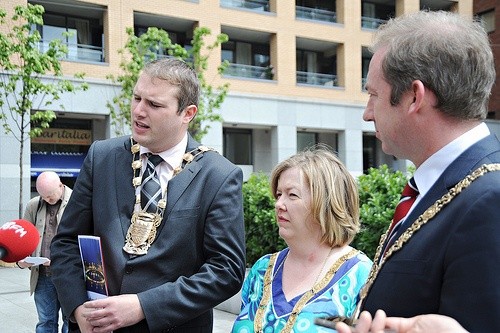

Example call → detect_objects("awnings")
31 152 85 177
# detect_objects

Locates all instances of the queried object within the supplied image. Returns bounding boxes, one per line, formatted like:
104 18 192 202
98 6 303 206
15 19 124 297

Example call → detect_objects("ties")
373 176 419 264
140 152 164 217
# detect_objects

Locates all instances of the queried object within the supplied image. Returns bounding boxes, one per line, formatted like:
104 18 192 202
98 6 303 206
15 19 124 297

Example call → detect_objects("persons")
332 10 500 333
227 149 373 333
51 57 246 333
18 172 73 333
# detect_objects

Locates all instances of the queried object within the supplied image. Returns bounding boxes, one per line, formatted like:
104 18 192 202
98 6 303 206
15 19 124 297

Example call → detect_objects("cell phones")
314 315 395 333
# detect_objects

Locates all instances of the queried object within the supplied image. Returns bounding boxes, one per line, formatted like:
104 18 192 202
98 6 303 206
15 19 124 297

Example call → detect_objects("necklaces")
349 163 500 325
313 248 333 286
121 141 209 255
